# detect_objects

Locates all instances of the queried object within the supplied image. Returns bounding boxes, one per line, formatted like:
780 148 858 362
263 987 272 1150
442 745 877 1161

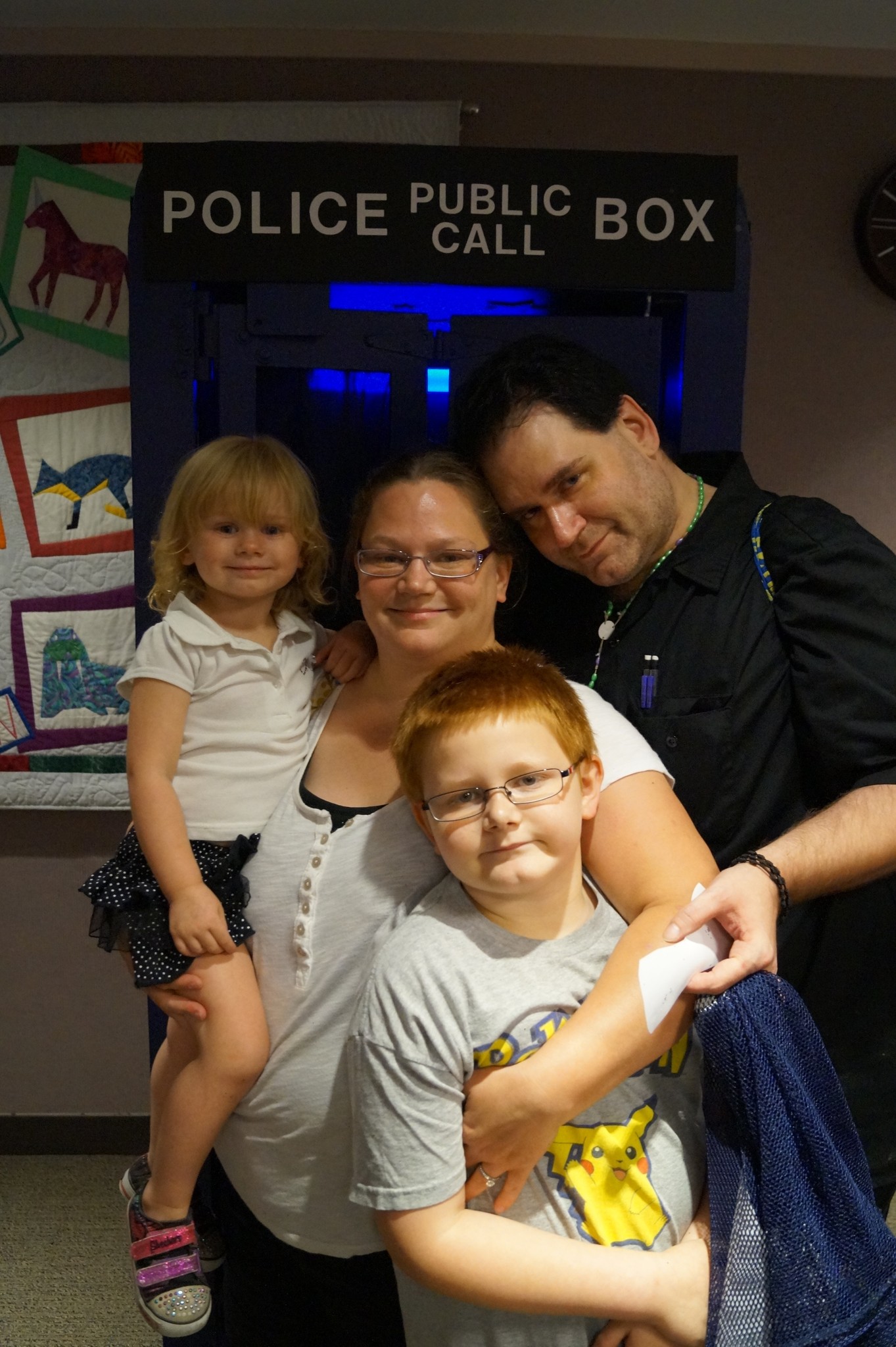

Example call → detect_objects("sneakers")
126 1194 211 1338
119 1153 151 1199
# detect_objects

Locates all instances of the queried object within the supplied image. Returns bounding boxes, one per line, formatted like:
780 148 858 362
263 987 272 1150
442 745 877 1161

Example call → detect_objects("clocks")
852 162 896 298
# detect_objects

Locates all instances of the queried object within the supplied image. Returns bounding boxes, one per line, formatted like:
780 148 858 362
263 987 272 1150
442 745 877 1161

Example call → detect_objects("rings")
476 1163 503 1188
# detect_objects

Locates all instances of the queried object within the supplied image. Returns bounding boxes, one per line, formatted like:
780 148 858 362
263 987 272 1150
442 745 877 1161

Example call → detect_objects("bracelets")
729 850 789 924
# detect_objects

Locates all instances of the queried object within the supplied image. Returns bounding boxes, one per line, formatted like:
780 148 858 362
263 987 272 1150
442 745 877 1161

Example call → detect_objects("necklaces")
588 466 705 691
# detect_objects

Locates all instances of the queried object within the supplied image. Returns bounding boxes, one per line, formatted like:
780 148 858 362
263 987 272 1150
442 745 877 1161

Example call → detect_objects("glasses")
356 544 496 578
421 755 588 821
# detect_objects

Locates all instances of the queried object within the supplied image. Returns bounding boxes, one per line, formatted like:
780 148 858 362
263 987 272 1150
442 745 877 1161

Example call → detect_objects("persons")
345 644 709 1347
111 446 732 1347
81 431 379 1338
477 360 896 1223
